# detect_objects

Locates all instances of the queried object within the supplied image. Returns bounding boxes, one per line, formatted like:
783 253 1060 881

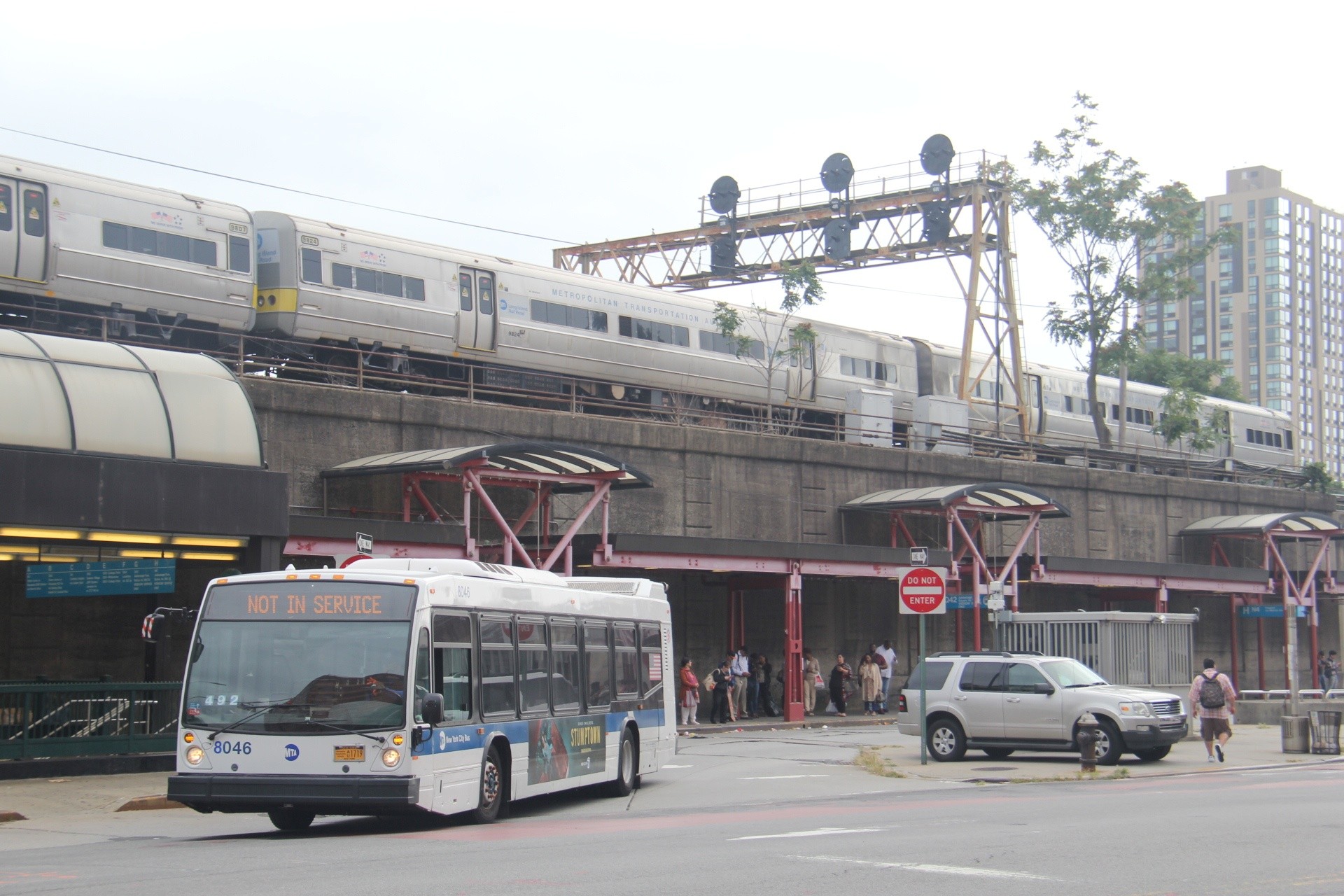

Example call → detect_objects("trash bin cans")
1308 710 1341 755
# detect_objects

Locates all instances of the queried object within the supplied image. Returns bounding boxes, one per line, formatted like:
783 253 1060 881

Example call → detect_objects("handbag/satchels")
814 671 825 690
825 699 838 713
876 689 885 702
770 701 780 714
702 668 720 691
843 670 859 692
683 690 696 707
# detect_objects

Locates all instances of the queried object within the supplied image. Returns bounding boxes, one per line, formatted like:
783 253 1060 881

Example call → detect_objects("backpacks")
1198 673 1224 708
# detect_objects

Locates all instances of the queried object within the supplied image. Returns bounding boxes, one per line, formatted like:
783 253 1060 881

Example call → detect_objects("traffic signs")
353 530 376 556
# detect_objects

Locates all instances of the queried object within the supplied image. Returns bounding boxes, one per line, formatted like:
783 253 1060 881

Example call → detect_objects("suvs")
898 650 1191 767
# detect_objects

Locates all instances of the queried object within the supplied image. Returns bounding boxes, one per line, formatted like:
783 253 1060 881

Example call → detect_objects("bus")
138 559 682 840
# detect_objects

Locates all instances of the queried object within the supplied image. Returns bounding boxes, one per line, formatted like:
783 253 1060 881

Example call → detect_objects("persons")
678 656 701 725
803 647 820 716
730 645 752 720
876 638 898 713
1317 649 1341 698
860 643 888 714
1189 658 1237 762
363 646 405 703
710 660 732 723
717 650 735 722
748 652 773 719
781 649 785 710
828 653 853 717
858 654 883 716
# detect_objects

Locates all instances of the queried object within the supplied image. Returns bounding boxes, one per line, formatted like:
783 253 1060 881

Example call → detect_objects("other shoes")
870 710 876 715
681 722 687 725
690 721 700 725
1208 756 1215 762
1215 744 1224 763
864 710 870 715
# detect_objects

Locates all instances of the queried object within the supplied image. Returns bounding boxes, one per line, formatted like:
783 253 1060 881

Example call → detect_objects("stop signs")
899 568 945 614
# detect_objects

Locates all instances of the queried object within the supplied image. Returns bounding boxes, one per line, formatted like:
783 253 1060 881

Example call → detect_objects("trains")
0 153 1310 482
